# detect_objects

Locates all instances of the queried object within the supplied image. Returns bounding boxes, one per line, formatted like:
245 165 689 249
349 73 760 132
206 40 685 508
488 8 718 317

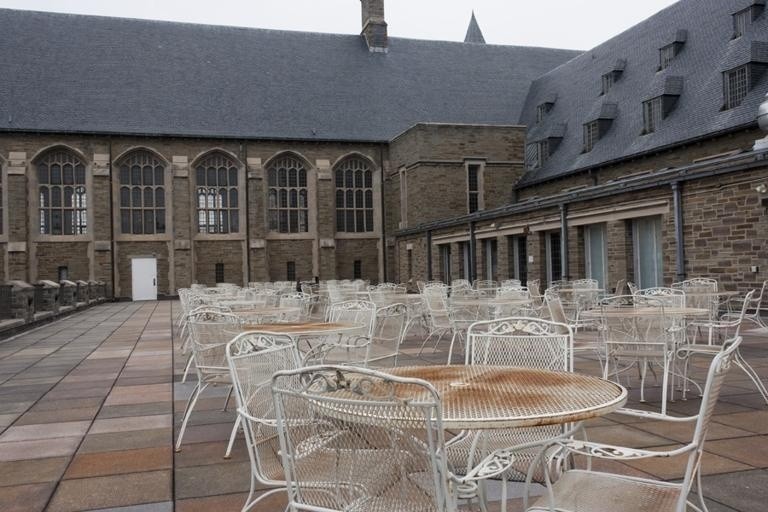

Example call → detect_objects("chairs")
172 276 767 512
